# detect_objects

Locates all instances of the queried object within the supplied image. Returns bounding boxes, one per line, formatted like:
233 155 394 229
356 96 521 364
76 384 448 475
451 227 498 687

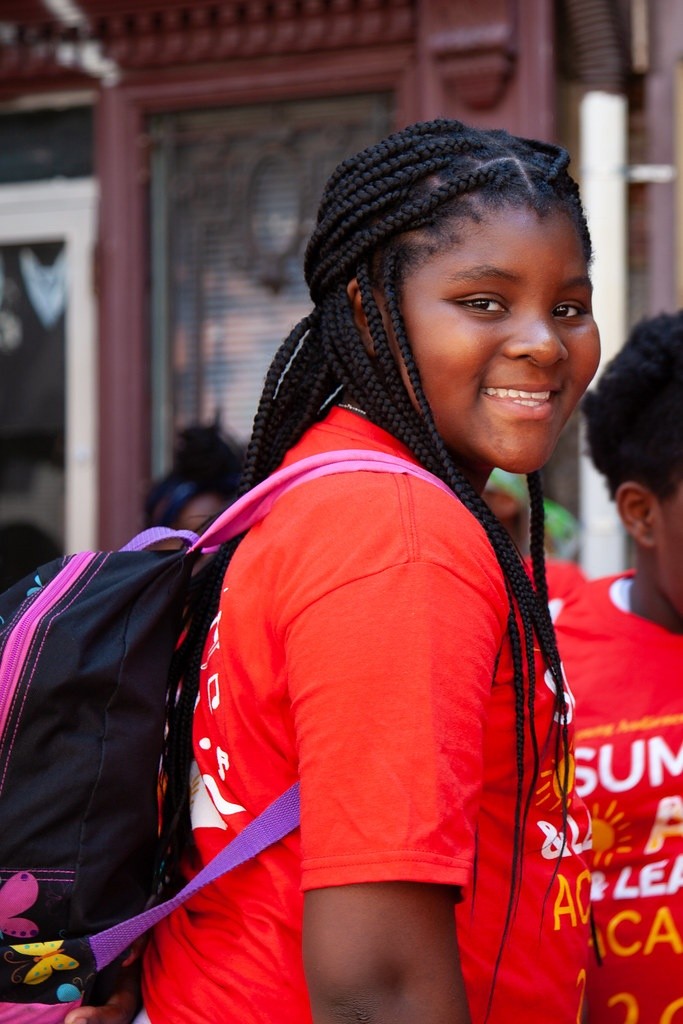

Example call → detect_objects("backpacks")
0 449 461 1024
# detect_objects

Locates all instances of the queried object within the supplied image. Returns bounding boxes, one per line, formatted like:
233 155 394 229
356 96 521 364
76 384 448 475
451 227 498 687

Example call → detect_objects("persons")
142 423 253 551
518 310 683 1024
131 118 602 1024
482 466 580 558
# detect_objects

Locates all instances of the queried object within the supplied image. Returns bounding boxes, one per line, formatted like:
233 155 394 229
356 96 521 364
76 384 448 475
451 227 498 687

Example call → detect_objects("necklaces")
338 403 366 415
